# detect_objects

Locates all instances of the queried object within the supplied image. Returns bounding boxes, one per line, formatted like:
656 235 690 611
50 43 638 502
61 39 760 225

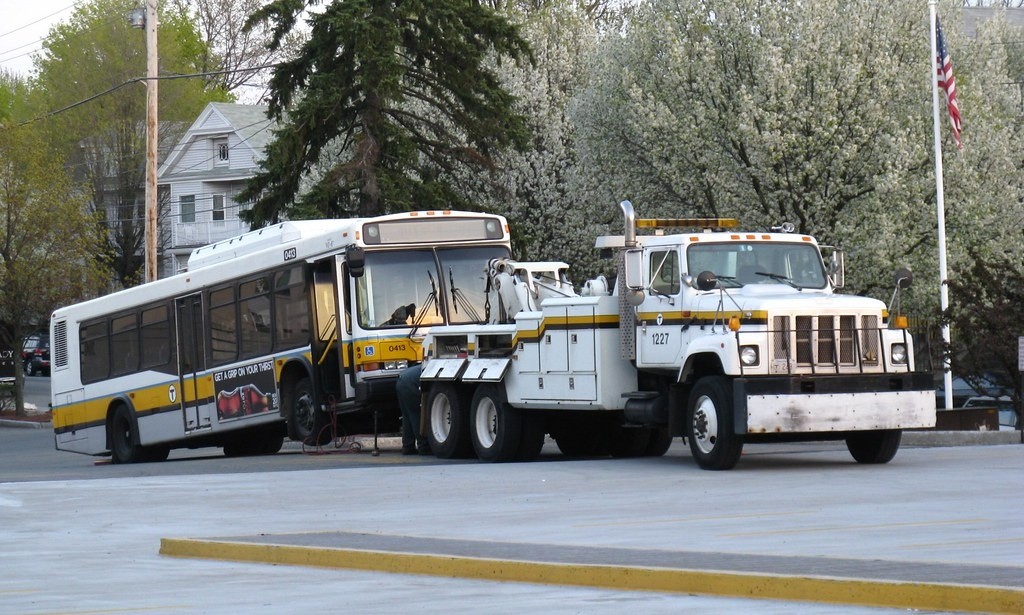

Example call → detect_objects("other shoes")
402 449 418 455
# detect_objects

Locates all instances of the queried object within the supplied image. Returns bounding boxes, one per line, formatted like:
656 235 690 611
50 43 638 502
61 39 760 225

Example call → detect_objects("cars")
2 334 50 377
935 368 1022 409
960 395 1019 430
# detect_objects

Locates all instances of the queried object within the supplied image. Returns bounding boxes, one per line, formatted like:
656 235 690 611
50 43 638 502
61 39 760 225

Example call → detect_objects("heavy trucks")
408 198 939 475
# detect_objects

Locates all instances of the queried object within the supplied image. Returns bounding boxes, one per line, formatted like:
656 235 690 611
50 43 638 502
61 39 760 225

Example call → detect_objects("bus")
48 208 515 458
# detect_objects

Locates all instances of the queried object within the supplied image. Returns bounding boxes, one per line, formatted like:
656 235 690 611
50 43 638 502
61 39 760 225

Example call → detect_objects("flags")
935 13 964 150
